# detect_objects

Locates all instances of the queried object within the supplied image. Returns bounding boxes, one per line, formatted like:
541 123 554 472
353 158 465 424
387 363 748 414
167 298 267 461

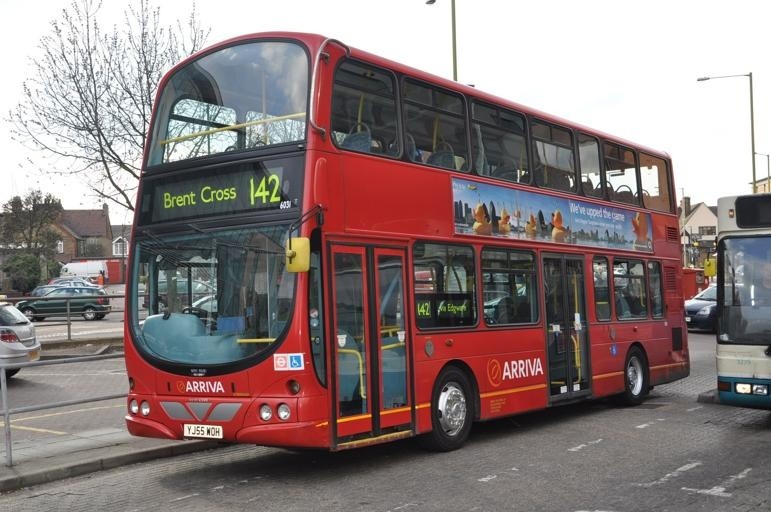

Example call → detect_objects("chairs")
493 292 660 324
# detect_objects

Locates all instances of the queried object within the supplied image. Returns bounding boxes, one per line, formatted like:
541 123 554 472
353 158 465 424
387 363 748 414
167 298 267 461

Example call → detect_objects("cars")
130 295 219 330
142 271 220 316
685 283 745 333
0 300 43 380
14 275 112 322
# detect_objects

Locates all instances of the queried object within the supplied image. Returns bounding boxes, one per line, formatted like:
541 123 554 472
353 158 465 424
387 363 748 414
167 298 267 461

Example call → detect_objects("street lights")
754 151 771 193
424 0 459 83
698 72 759 193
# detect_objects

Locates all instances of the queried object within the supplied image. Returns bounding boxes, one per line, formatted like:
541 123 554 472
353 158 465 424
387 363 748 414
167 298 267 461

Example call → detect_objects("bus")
122 31 695 457
703 192 771 411
